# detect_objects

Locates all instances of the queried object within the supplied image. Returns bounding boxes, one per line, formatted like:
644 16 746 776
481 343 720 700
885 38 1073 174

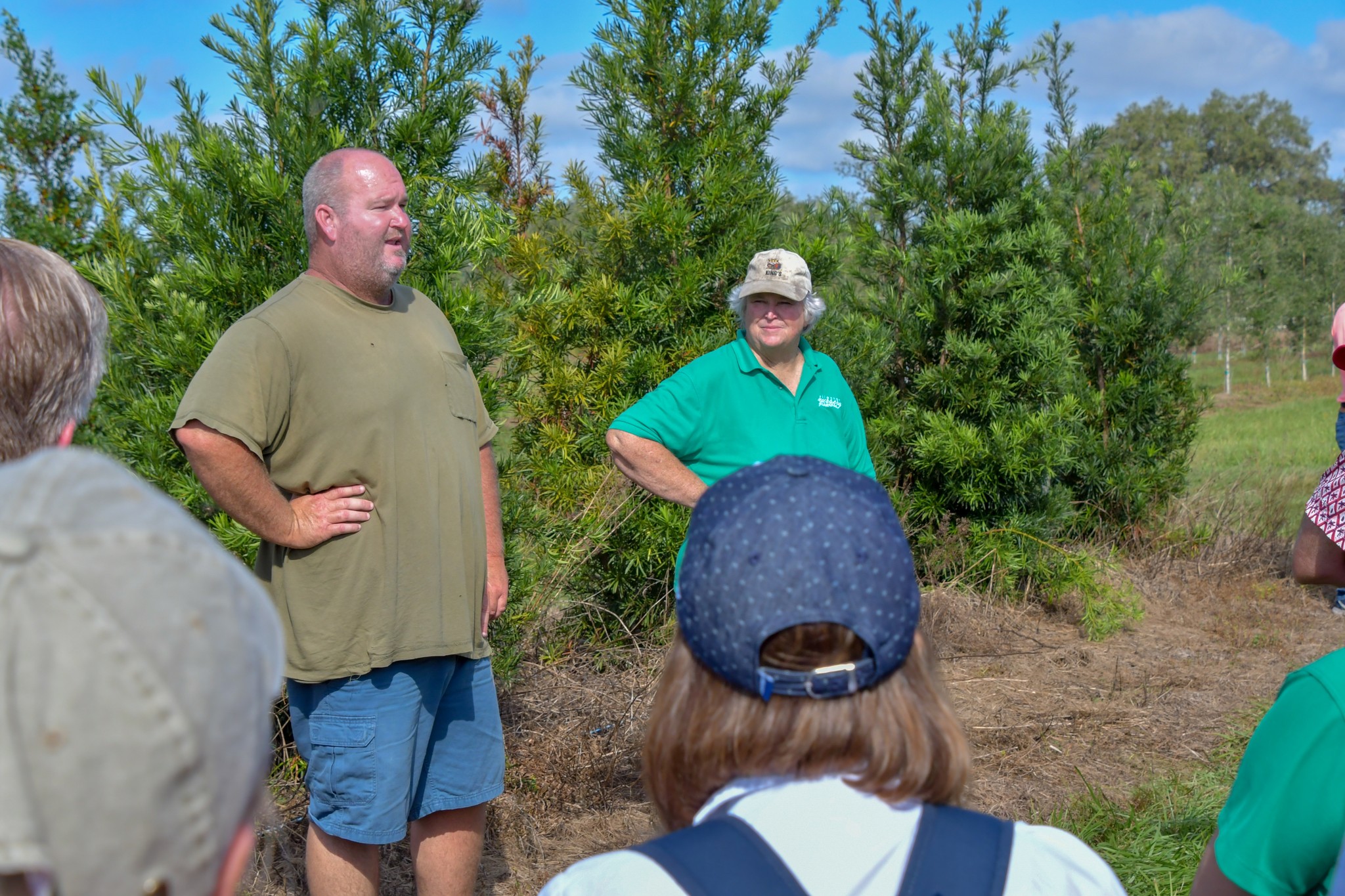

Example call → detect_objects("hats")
739 247 814 302
673 454 922 701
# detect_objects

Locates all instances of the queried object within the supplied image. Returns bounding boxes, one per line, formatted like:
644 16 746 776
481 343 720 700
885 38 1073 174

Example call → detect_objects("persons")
167 147 509 896
0 235 109 467
605 249 877 593
1332 300 1345 618
0 446 287 896
527 456 1133 896
1190 644 1343 896
1290 344 1345 589
